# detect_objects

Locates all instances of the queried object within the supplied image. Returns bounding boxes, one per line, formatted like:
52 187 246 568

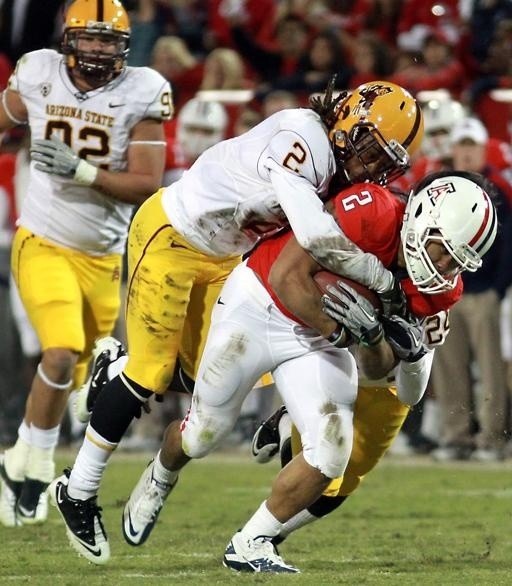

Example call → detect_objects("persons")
1 0 512 575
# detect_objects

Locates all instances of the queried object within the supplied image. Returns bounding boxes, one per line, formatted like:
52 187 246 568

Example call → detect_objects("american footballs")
313 271 383 309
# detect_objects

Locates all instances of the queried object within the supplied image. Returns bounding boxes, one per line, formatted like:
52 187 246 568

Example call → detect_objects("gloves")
318 279 389 349
383 309 427 362
29 132 98 187
379 278 411 321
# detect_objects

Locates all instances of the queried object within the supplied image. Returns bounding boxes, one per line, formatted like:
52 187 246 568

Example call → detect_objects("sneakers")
387 431 512 463
0 454 57 529
120 458 180 548
72 334 125 423
249 402 291 464
44 468 111 569
221 527 303 577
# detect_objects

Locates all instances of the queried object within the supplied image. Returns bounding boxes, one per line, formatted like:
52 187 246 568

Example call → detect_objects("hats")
399 20 459 56
418 73 493 160
397 169 497 297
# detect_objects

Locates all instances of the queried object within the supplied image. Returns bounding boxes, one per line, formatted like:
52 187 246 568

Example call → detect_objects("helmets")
59 0 132 91
329 78 426 190
174 96 229 165
